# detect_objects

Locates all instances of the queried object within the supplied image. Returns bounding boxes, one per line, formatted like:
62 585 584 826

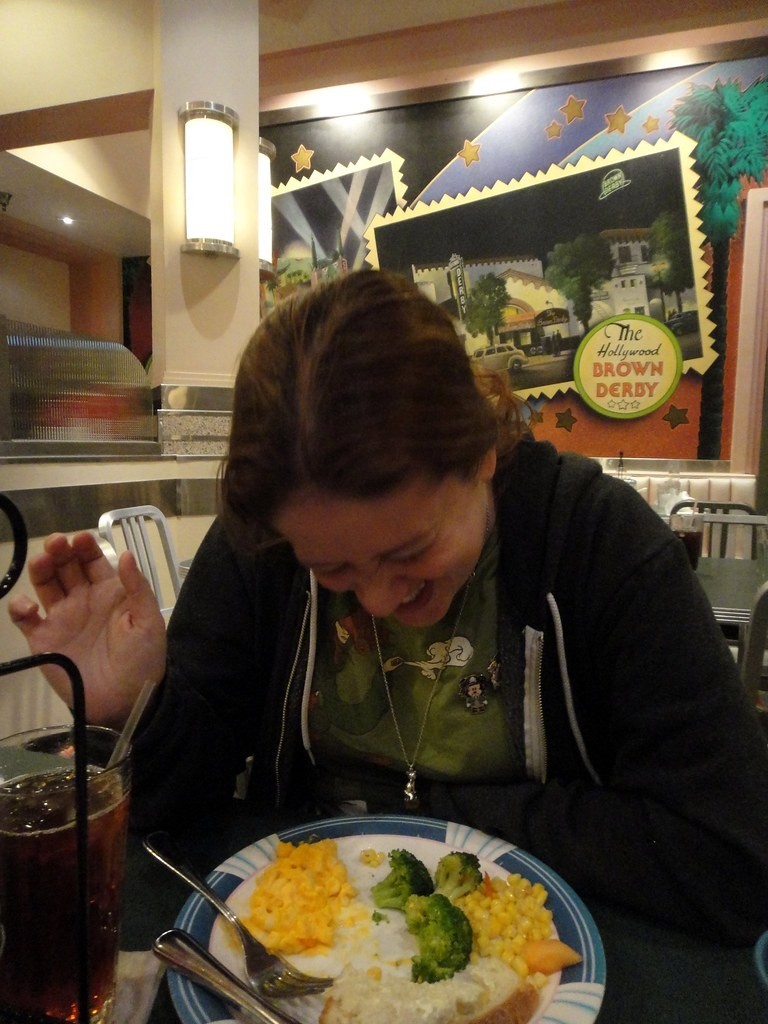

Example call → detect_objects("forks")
140 830 336 1000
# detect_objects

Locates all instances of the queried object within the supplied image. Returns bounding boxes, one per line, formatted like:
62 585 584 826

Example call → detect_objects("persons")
552 329 562 356
8 271 768 952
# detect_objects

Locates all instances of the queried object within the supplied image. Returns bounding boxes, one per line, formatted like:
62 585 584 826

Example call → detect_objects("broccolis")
372 847 487 983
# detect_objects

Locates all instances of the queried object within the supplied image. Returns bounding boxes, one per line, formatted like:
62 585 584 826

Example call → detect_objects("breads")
318 955 543 1024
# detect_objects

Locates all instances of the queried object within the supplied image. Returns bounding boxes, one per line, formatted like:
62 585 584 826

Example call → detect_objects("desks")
693 557 768 695
0 745 768 1024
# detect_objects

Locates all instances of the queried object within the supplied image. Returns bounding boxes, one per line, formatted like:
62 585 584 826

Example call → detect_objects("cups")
0 723 132 1024
668 513 704 572
622 478 638 491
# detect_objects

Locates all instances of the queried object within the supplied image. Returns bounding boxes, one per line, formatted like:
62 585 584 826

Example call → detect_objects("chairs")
663 501 768 731
86 503 179 628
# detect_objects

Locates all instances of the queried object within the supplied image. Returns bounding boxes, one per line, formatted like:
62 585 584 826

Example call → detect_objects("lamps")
258 136 275 280
179 101 240 259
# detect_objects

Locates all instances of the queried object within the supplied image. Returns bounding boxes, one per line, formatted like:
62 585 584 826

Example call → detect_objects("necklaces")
369 583 470 808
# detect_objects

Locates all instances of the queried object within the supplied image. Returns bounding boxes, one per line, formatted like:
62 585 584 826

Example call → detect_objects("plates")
167 815 609 1024
